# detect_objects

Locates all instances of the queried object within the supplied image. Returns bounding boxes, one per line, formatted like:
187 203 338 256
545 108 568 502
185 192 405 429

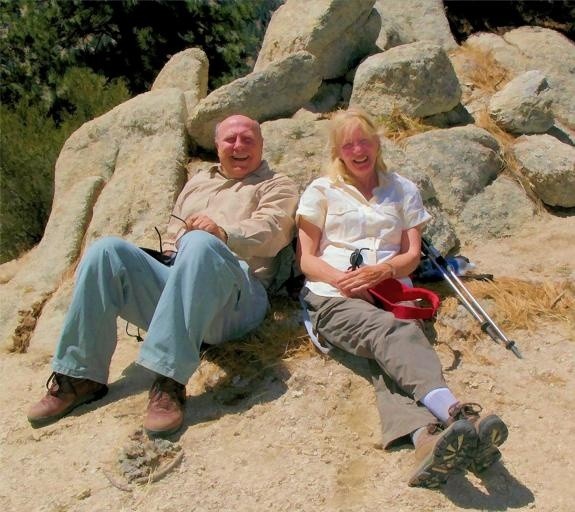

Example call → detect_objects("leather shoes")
143 376 186 436
28 373 107 422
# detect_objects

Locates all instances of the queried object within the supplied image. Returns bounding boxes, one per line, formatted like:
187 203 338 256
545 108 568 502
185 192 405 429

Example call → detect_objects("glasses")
347 248 369 271
155 215 188 264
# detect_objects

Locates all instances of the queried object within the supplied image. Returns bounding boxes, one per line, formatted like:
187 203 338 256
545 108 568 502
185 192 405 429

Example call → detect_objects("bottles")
441 257 478 278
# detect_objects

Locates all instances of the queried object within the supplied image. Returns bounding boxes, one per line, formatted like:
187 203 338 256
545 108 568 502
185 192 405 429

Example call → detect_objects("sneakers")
445 402 507 470
409 418 477 487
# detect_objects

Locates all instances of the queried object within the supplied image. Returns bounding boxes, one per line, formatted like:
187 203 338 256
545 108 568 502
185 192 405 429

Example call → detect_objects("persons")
27 112 300 439
292 108 509 490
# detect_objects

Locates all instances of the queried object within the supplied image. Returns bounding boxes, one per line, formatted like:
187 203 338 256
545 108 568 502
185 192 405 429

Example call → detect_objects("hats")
368 278 440 319
